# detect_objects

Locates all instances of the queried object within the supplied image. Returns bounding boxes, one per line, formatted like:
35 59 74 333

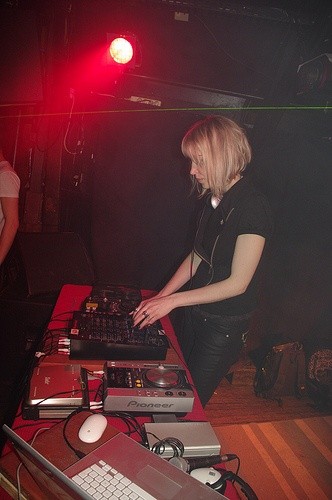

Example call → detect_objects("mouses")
78 413 107 444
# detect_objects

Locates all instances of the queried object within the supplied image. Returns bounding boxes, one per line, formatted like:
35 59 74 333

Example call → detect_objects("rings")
142 312 148 317
134 307 139 312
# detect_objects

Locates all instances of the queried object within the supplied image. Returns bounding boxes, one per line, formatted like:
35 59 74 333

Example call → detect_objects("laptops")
2 424 231 500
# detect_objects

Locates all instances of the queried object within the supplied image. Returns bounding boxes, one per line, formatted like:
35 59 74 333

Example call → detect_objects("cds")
146 368 180 388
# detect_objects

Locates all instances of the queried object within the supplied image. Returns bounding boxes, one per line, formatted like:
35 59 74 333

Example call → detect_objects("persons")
0 132 21 267
128 112 276 416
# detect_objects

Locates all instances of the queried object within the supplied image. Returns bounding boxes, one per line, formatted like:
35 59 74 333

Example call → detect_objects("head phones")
189 466 258 500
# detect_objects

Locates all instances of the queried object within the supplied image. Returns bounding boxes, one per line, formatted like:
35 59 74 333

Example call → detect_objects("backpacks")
251 341 312 407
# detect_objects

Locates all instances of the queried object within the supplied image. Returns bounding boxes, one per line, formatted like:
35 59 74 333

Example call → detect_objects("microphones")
169 454 238 472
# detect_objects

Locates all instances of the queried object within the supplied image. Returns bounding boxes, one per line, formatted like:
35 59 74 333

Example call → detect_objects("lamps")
104 21 149 74
294 52 332 103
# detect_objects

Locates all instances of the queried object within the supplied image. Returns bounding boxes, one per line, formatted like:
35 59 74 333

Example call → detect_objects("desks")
0 285 240 500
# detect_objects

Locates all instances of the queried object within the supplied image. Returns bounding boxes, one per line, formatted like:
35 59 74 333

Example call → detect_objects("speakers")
15 230 96 299
